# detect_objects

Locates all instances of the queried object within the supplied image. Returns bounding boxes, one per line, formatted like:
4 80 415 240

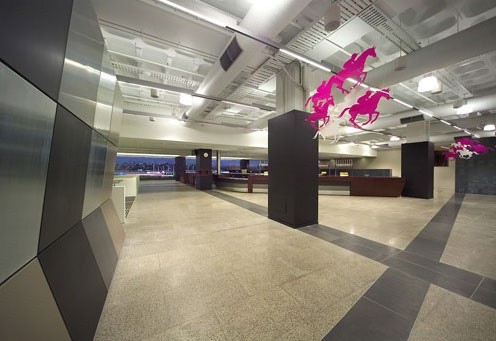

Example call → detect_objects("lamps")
452 85 464 108
149 117 156 122
150 88 160 100
417 71 439 93
430 81 443 95
393 16 408 72
324 0 341 32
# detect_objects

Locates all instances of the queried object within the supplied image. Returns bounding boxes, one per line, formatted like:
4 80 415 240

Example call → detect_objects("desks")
248 174 405 197
